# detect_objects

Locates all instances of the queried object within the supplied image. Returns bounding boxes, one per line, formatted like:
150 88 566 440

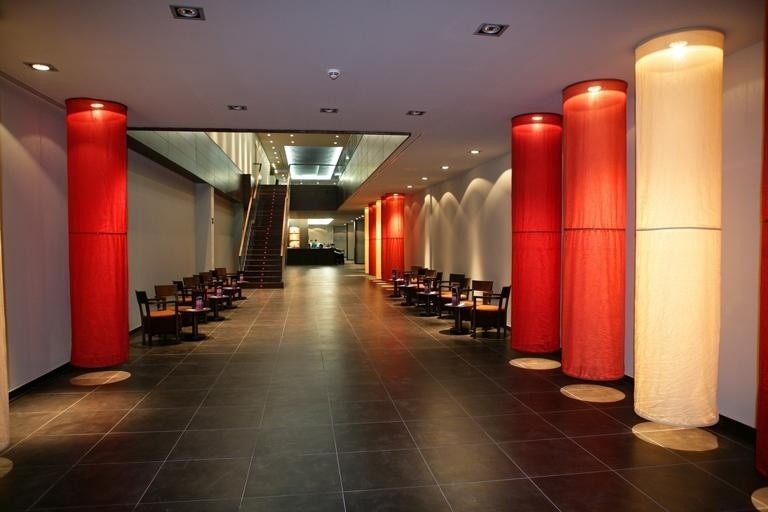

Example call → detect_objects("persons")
309 240 313 247
312 239 317 248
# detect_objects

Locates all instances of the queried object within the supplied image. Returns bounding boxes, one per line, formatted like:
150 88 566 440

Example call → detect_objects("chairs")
388 266 512 340
134 267 251 345
